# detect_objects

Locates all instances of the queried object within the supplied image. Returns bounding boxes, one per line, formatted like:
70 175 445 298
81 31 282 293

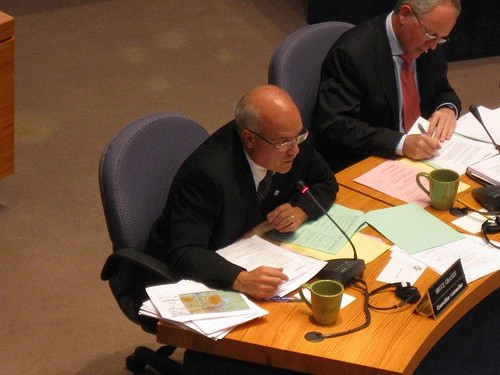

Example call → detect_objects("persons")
134 84 339 375
307 0 463 174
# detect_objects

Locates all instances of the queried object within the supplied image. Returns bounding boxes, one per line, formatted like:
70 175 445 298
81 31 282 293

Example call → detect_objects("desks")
156 182 500 375
332 154 500 243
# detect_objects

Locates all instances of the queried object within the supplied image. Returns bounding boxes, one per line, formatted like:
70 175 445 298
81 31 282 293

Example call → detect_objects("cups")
415 168 459 211
299 279 344 326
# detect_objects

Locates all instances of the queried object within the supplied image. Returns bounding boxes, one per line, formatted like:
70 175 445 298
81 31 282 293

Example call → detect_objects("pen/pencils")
456 199 481 213
260 296 299 301
417 122 430 137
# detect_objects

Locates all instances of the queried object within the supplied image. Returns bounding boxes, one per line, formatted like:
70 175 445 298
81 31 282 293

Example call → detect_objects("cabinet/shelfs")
0 10 16 179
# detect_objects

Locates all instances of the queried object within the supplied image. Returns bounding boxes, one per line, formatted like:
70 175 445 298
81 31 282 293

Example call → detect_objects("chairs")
267 21 358 135
100 111 211 375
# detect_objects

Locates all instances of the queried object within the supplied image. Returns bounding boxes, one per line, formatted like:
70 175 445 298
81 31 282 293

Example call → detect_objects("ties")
252 169 275 229
397 53 422 134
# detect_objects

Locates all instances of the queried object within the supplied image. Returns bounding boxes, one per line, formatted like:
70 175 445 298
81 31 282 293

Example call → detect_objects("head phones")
482 216 500 249
367 281 421 310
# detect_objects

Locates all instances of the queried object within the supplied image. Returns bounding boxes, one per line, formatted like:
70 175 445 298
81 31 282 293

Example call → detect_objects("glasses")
403 3 450 46
245 127 309 152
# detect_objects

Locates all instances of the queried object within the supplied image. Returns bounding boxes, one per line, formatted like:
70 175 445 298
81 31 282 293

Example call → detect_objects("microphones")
297 180 366 287
469 105 500 154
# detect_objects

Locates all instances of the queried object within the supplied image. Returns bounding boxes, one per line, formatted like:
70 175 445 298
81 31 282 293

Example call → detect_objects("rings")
291 214 296 221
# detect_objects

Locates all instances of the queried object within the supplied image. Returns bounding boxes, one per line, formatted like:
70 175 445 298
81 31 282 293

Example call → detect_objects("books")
466 152 499 189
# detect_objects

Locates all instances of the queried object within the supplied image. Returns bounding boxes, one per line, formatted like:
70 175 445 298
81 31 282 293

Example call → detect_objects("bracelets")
444 103 454 109
